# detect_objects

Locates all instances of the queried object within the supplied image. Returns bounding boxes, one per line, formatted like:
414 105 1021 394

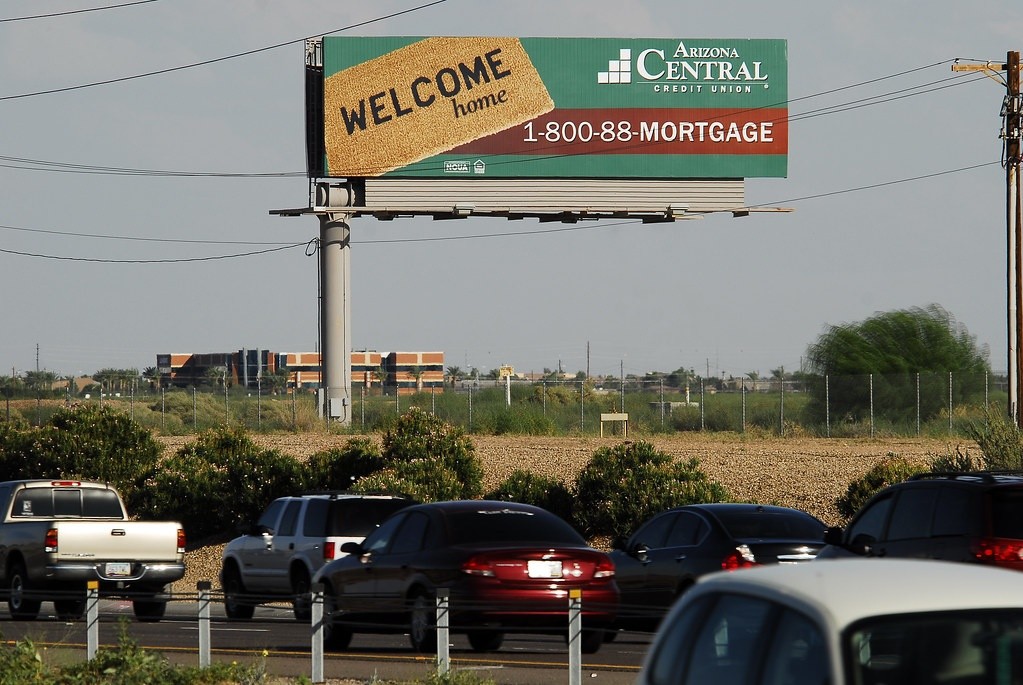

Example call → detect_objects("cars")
609 502 845 633
314 499 619 654
636 559 1022 685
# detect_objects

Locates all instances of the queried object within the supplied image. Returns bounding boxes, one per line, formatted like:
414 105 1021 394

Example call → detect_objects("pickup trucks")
1 481 188 623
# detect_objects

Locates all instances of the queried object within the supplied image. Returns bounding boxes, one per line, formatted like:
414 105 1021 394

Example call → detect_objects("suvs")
836 470 1023 572
218 490 417 625
